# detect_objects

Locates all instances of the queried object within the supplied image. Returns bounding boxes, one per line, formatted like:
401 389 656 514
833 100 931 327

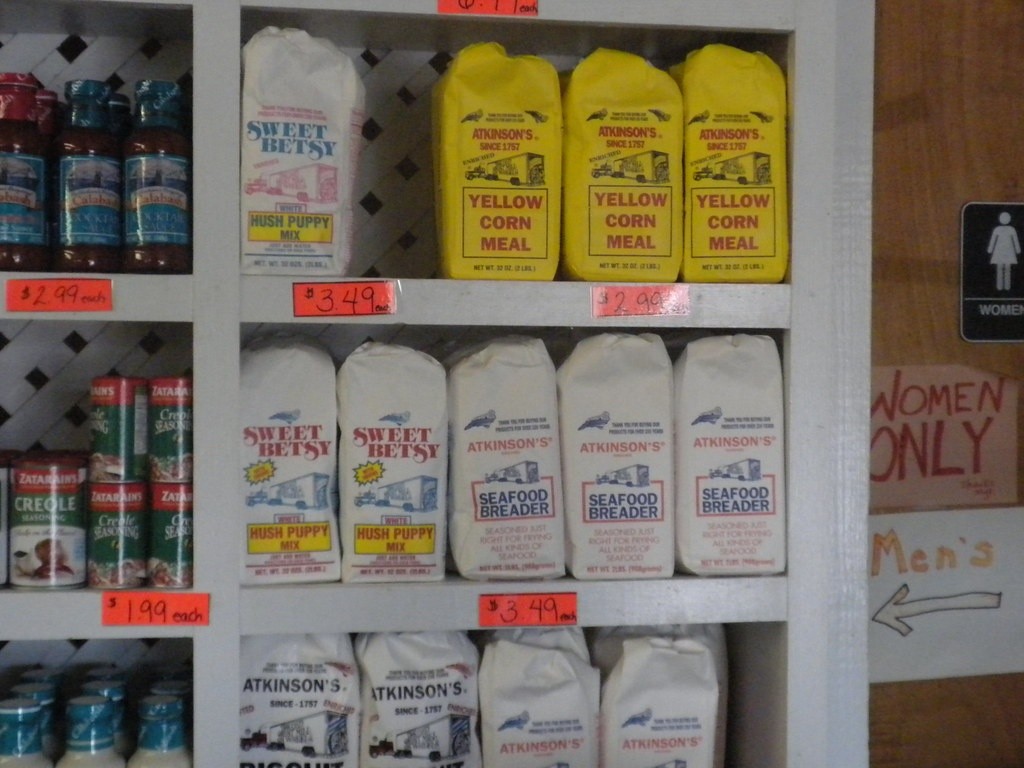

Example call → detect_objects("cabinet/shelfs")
0 0 875 768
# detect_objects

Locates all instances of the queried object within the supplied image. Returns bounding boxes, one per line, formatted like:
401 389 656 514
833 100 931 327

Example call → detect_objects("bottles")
59 79 133 272
0 665 192 768
123 79 193 274
0 72 60 272
